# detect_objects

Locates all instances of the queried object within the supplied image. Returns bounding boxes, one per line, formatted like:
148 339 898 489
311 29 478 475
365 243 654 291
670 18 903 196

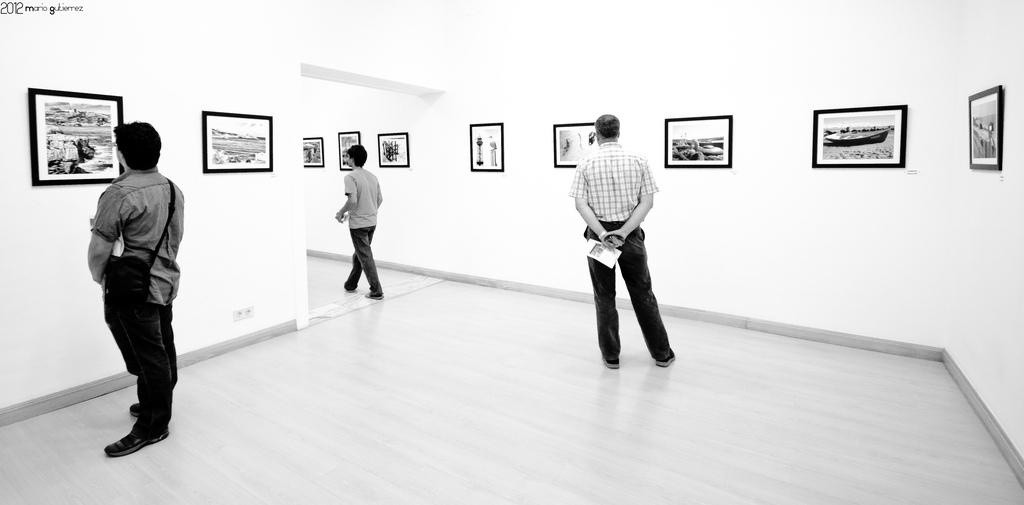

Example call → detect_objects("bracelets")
599 230 607 240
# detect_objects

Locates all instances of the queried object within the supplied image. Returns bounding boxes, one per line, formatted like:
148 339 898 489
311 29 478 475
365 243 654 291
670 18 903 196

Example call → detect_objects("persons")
88 121 184 458
334 145 384 300
487 135 497 166
568 114 675 369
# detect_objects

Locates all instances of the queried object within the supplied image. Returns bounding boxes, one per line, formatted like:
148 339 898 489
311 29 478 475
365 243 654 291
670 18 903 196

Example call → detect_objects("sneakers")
602 356 619 368
366 291 383 300
345 283 356 291
655 348 674 367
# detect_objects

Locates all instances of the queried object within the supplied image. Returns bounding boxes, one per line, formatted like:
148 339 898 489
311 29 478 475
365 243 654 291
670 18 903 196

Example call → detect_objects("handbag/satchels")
104 255 149 324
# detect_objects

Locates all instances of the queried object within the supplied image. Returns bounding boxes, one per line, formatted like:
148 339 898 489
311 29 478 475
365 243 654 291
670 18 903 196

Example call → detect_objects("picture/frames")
28 88 123 188
378 133 409 167
202 111 273 171
338 131 360 170
469 123 504 172
968 85 1003 170
303 137 324 167
665 115 733 169
812 104 908 168
553 123 597 169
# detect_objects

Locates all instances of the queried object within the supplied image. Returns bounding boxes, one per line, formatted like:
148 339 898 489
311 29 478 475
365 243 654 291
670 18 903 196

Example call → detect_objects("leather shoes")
104 427 169 456
130 403 141 417
